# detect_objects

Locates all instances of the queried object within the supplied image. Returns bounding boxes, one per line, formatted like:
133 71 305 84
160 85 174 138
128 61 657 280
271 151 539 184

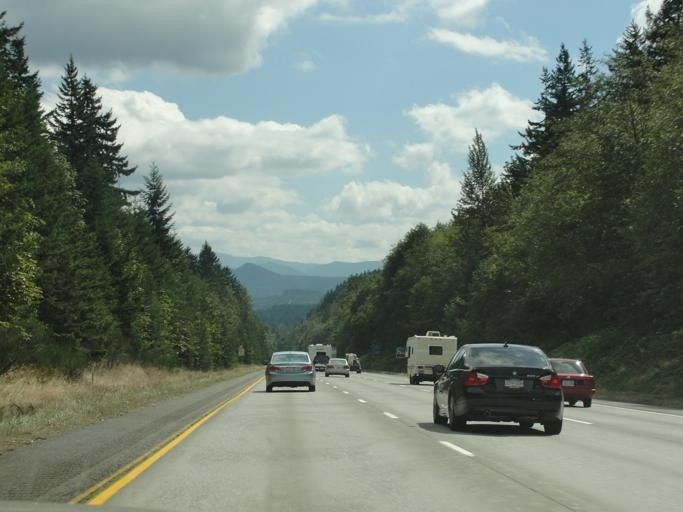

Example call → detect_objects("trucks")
405 329 457 384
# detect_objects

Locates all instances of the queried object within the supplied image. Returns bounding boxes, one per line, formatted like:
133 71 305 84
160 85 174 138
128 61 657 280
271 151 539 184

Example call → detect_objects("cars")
549 355 594 409
265 341 364 391
434 341 563 435
